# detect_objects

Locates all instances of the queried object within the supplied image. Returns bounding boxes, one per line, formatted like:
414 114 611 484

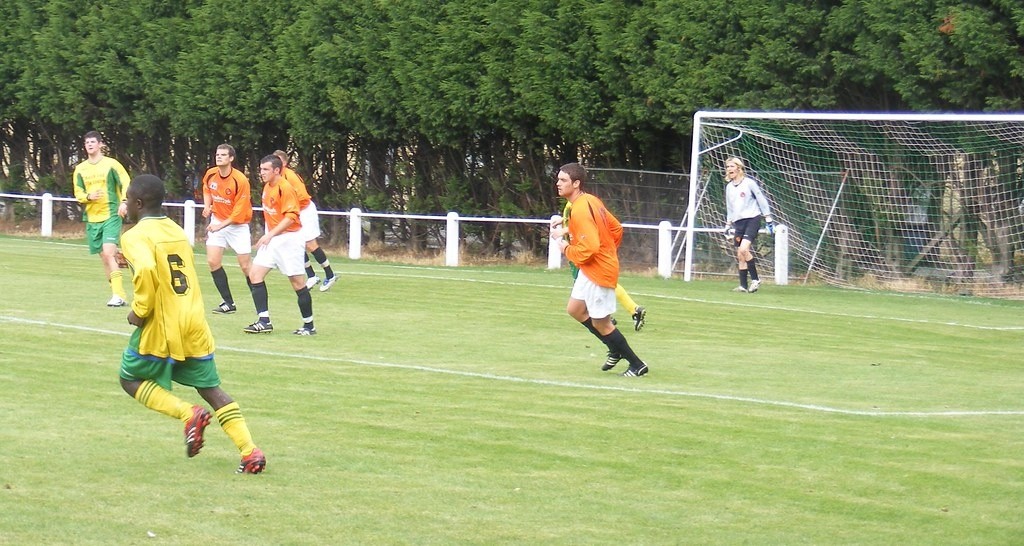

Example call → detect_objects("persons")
244 154 317 336
549 163 650 380
724 158 774 294
202 143 253 314
114 175 268 475
272 149 339 292
74 132 131 305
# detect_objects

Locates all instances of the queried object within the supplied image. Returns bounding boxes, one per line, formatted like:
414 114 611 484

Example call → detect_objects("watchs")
87 194 92 201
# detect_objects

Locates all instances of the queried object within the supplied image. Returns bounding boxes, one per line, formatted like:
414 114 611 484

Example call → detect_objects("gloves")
725 224 732 234
765 221 776 235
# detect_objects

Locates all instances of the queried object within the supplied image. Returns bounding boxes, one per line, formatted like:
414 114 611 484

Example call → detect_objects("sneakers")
235 448 266 474
749 278 762 292
185 405 213 457
731 285 748 292
243 320 273 334
107 295 128 306
294 326 316 336
618 361 649 376
212 301 236 313
306 273 321 290
601 351 622 370
319 272 339 292
632 306 647 331
